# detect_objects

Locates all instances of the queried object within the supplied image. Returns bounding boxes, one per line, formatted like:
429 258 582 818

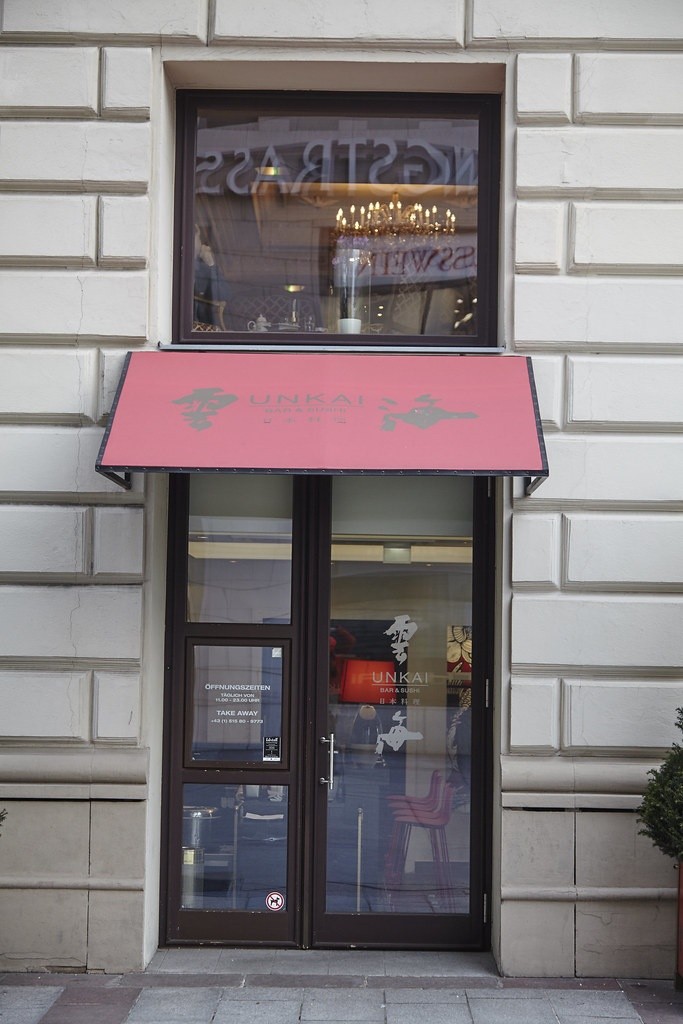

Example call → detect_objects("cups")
338 318 359 334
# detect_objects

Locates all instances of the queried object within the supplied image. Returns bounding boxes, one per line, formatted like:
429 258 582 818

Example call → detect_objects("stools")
387 767 456 907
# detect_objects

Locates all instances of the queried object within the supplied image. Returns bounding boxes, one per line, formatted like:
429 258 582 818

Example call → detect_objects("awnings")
94 352 550 497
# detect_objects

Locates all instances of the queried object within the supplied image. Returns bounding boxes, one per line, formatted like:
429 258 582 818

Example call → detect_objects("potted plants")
637 707 683 996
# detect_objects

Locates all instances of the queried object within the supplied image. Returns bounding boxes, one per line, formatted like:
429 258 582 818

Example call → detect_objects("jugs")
247 314 270 332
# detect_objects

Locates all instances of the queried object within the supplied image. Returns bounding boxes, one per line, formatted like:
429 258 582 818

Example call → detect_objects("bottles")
303 308 316 332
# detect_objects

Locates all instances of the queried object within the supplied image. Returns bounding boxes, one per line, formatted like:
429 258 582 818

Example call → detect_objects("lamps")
336 194 457 247
383 543 413 564
